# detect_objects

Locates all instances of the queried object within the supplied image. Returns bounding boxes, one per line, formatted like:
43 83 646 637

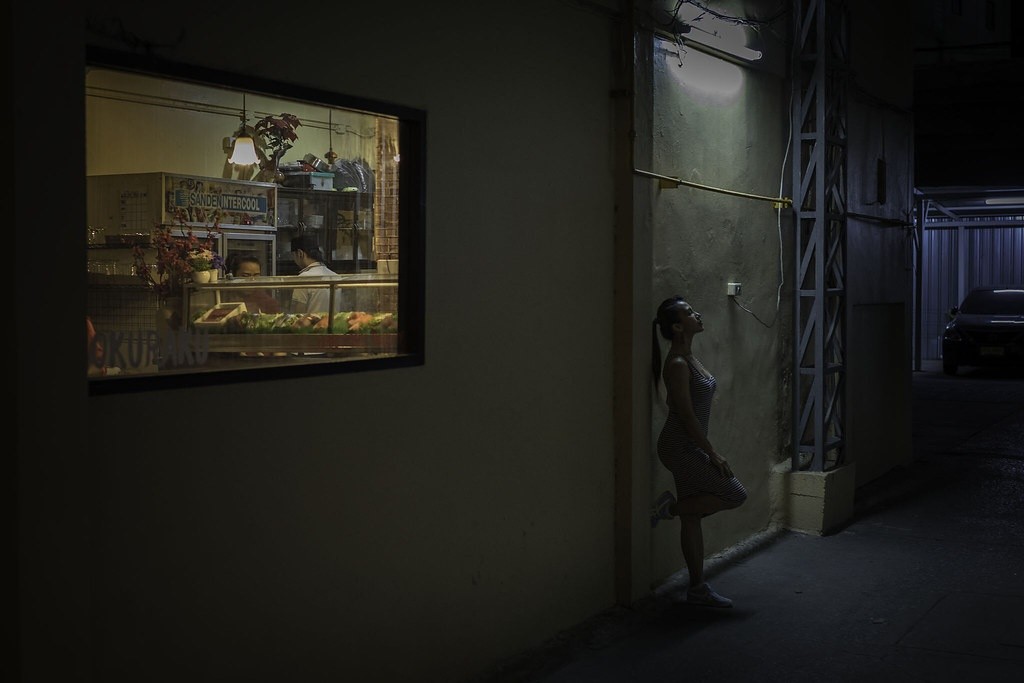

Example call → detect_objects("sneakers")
639 489 676 530
687 583 733 611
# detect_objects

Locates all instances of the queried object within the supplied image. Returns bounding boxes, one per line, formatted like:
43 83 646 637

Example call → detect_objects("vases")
191 268 219 285
263 161 286 183
157 297 184 351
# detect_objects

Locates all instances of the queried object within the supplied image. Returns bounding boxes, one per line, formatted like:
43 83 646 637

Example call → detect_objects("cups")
87 256 135 275
87 223 104 245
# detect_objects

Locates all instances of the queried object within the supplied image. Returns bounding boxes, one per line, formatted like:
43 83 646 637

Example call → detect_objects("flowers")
122 203 228 300
185 250 214 270
252 111 302 170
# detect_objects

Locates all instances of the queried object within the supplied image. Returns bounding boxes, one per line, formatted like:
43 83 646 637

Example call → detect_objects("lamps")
678 23 762 63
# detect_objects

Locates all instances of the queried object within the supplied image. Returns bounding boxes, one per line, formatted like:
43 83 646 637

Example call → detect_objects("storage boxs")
283 170 336 192
193 300 249 334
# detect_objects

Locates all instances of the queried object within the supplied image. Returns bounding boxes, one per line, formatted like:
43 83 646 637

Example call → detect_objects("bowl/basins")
305 214 323 226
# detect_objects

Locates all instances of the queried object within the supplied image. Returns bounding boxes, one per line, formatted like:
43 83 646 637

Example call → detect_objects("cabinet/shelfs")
275 187 379 275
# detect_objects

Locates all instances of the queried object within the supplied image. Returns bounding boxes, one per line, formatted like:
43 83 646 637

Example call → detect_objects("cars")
941 285 1024 372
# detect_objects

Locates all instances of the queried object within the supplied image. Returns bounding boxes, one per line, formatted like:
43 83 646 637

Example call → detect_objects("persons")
651 296 749 606
225 253 282 313
288 237 343 313
